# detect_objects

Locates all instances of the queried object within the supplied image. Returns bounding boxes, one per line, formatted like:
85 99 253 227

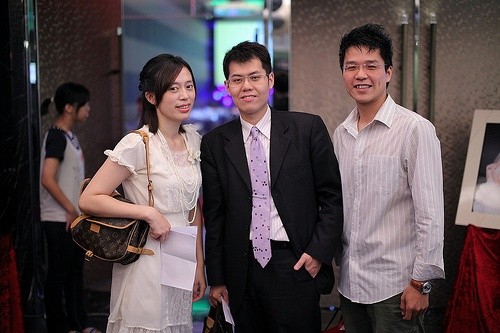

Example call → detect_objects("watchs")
410 279 433 294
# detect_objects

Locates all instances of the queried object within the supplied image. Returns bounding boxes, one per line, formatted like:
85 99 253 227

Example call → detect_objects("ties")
249 128 272 269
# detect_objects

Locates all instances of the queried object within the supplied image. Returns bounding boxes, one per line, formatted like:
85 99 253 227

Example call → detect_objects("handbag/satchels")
70 130 155 265
202 301 232 333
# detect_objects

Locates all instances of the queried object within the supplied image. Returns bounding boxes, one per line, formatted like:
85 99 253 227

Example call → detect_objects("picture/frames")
454 110 500 232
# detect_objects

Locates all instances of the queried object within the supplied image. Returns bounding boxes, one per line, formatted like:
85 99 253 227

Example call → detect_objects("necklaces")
157 128 199 223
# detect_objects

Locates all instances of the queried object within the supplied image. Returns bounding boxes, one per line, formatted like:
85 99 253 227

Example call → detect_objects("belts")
271 240 291 250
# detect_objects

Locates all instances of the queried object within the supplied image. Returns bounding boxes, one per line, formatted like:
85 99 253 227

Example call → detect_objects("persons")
39 82 99 333
78 53 206 333
332 23 446 333
200 40 343 333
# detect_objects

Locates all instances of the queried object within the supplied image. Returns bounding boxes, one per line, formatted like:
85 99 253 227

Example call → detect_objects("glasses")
343 64 384 72
228 73 267 87
